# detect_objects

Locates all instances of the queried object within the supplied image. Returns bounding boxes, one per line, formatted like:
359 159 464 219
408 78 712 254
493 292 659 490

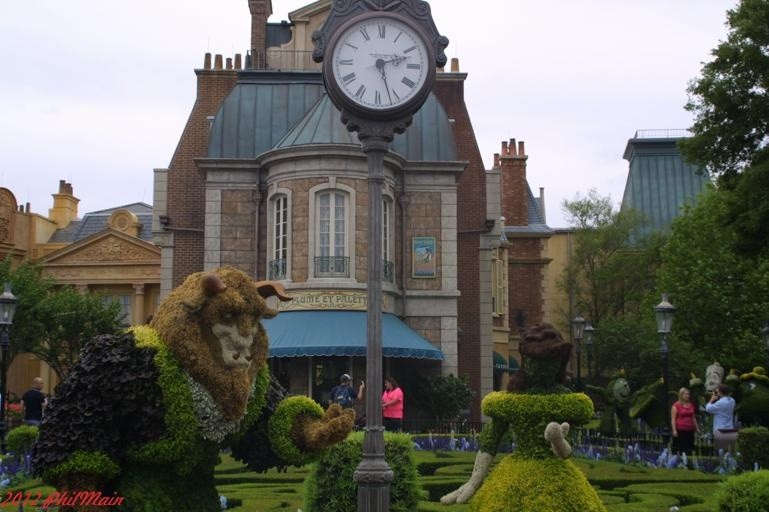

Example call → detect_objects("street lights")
0 286 19 436
581 319 597 385
651 292 678 451
571 312 586 392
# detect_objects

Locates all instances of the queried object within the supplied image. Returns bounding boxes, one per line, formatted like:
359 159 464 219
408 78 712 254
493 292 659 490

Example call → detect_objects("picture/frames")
412 236 436 278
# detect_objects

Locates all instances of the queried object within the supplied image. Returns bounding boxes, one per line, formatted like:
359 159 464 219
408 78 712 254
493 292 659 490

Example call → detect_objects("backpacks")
333 386 352 405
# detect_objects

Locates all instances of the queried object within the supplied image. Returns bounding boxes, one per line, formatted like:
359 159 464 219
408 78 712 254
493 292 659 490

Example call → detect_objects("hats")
340 374 352 382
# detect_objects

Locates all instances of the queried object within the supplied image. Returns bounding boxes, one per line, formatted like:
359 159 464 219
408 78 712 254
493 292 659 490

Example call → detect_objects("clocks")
323 11 437 121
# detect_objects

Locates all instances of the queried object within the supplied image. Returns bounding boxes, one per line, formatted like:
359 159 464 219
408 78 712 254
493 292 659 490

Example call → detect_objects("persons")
670 387 700 455
327 373 364 411
705 384 737 436
19 377 49 429
381 374 403 433
